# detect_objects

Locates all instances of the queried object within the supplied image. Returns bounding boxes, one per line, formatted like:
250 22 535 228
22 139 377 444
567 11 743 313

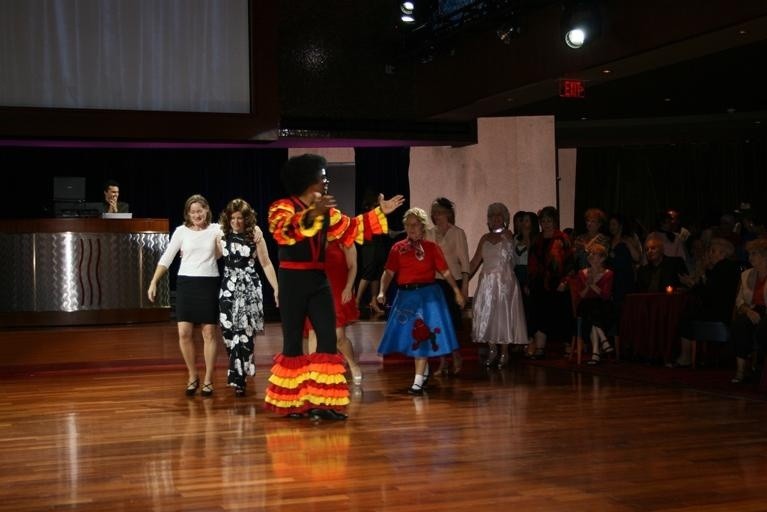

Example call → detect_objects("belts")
398 282 437 291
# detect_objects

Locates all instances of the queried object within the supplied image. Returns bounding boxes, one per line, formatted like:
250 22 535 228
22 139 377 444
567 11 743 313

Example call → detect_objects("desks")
619 288 690 366
0 216 171 326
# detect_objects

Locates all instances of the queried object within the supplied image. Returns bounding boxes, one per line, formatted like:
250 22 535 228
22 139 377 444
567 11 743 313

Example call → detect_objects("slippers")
666 357 693 368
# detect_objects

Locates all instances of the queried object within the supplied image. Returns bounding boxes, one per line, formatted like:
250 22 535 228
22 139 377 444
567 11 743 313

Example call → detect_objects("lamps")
564 25 590 50
400 1 416 24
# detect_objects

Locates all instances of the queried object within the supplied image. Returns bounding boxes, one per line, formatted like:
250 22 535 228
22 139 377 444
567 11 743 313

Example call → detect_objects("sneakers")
432 369 449 378
454 371 466 376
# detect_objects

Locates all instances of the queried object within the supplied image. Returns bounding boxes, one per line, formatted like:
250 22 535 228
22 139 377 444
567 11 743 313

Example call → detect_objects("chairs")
566 275 619 366
691 268 747 366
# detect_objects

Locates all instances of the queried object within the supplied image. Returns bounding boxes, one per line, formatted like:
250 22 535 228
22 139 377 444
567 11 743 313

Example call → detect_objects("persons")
302 241 363 388
355 194 405 316
264 154 406 421
180 199 279 395
377 207 466 394
148 195 263 396
102 181 130 212
470 201 762 393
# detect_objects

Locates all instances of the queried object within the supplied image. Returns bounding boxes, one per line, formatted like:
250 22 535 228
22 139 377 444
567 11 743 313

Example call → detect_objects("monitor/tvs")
53 177 86 202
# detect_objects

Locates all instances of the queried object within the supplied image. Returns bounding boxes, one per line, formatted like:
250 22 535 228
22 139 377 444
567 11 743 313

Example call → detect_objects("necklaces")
488 225 507 237
433 226 445 243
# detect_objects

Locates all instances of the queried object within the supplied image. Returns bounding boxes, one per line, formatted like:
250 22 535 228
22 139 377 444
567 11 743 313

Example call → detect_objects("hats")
437 197 454 208
734 199 756 215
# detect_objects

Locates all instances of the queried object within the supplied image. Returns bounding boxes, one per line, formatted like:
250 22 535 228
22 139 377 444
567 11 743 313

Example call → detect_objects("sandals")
350 363 363 386
600 338 615 356
587 352 604 367
732 371 745 384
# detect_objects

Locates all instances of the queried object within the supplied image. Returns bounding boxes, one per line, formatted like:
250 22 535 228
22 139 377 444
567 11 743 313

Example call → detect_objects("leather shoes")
312 408 349 422
287 412 305 418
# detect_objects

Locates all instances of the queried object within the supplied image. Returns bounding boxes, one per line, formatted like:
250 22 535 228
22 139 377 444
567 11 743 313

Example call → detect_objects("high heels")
202 382 214 396
186 378 199 395
369 303 385 313
484 350 497 367
408 385 424 395
226 379 238 388
235 381 248 398
498 353 510 369
423 362 431 384
